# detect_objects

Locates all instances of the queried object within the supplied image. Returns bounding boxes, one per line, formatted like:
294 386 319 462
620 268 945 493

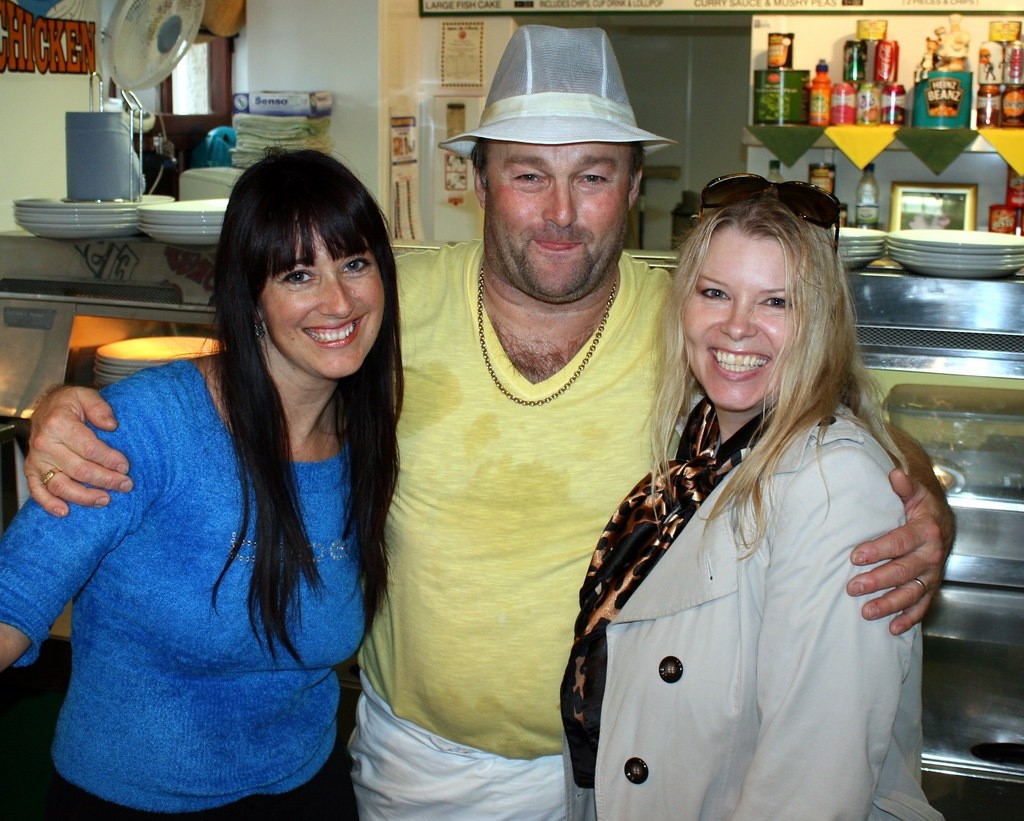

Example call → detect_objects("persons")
0 149 405 821
25 24 955 821
561 173 949 821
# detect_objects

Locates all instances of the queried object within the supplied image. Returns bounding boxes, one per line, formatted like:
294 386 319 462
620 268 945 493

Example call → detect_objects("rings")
913 578 926 598
40 469 62 490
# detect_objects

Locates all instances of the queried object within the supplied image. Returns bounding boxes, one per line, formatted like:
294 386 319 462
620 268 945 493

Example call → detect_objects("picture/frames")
888 179 979 232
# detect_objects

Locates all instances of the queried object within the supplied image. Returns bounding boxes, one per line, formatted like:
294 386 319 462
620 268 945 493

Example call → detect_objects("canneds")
766 32 795 70
827 18 907 125
807 162 847 226
988 162 1024 238
974 19 1024 131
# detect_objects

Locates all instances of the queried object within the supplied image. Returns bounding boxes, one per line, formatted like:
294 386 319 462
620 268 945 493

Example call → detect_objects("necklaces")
479 266 617 406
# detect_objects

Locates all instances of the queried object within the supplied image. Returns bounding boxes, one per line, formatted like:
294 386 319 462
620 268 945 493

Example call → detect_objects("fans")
100 0 206 92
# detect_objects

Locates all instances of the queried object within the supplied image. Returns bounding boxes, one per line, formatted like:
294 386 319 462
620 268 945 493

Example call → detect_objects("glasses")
696 172 842 253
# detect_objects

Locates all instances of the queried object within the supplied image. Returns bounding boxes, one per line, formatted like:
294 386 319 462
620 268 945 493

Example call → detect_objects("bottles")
767 161 782 184
810 59 830 127
855 163 879 230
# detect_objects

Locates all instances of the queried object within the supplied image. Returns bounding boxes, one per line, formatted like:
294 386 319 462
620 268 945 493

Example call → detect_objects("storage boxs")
232 90 332 119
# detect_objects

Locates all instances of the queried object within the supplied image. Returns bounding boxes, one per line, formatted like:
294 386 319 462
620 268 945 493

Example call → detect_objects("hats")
438 24 679 158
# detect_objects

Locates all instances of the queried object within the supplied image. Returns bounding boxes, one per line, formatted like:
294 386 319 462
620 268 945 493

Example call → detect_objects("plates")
137 198 229 245
887 230 1024 280
12 195 175 240
833 227 887 271
94 337 226 390
